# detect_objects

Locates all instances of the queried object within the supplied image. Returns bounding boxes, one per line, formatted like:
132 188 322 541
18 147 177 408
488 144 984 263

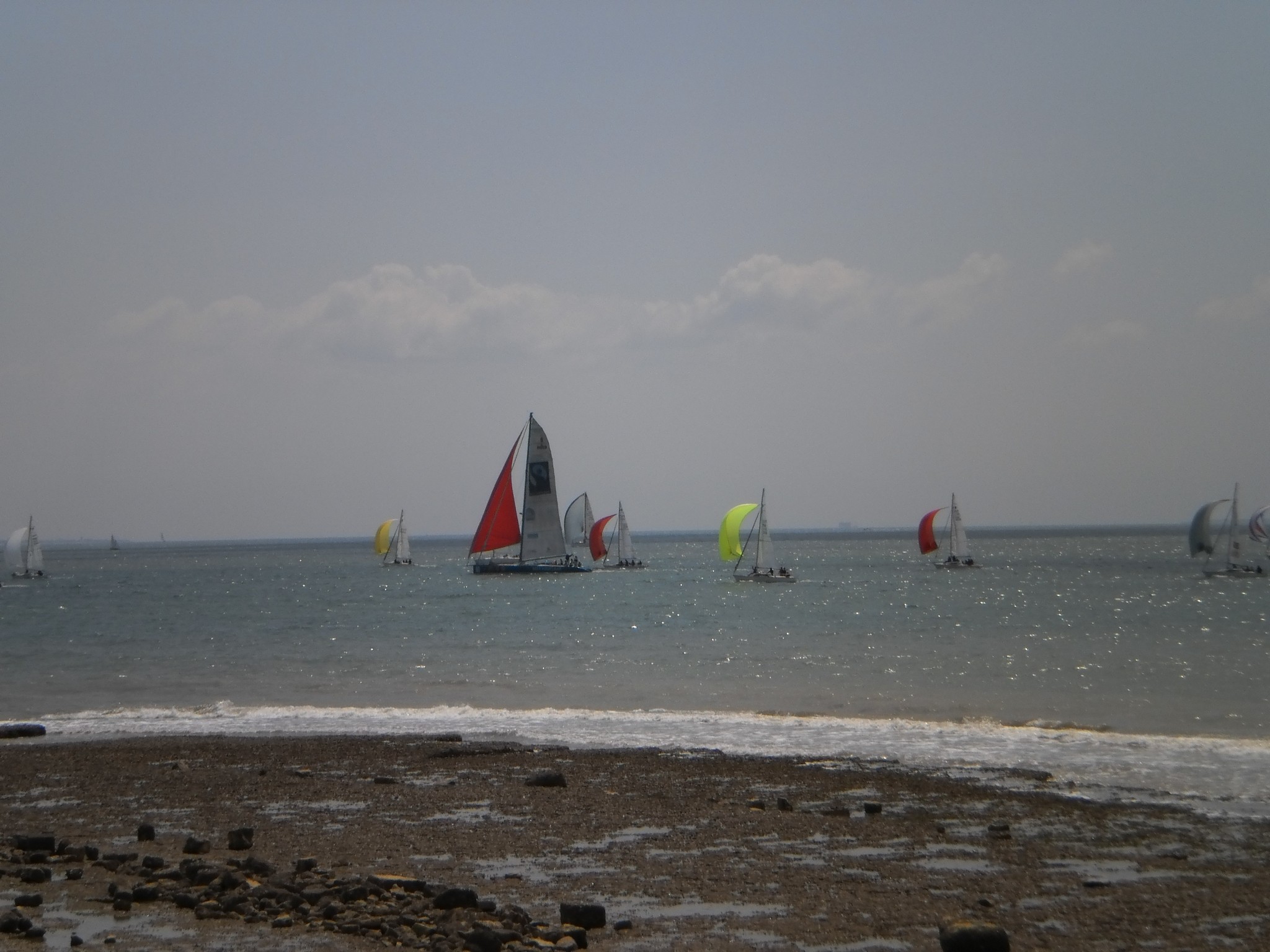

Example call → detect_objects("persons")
555 554 582 567
948 555 974 565
1233 564 1262 574
620 559 642 566
394 559 412 564
748 566 786 576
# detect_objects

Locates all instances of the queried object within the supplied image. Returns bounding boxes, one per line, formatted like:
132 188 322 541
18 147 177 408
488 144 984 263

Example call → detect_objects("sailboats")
1188 481 1267 578
465 411 595 577
7 515 48 579
109 534 121 550
916 490 984 569
374 509 415 567
1248 505 1270 558
718 488 796 583
589 491 646 570
